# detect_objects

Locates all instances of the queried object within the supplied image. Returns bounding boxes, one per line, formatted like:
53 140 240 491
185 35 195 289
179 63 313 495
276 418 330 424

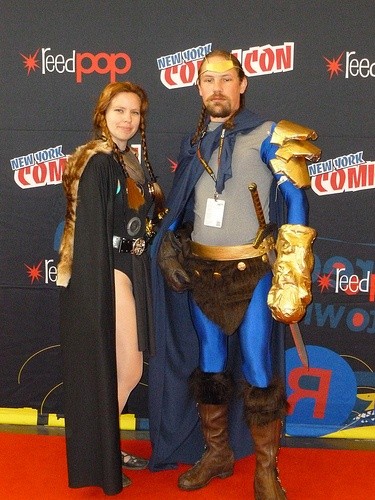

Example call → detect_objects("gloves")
158 229 194 293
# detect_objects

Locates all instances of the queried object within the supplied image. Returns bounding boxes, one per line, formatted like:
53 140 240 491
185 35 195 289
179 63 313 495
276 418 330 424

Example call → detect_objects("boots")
237 371 292 500
177 368 239 490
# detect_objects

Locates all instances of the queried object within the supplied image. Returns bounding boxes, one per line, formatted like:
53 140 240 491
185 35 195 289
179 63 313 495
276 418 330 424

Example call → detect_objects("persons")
53 81 170 496
149 47 318 500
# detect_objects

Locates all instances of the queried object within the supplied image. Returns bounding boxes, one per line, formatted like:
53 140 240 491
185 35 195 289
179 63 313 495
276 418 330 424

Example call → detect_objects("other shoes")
122 451 149 470
122 472 132 487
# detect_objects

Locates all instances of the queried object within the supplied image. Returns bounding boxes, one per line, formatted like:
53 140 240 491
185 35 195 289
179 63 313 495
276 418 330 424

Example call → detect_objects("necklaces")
197 122 226 202
